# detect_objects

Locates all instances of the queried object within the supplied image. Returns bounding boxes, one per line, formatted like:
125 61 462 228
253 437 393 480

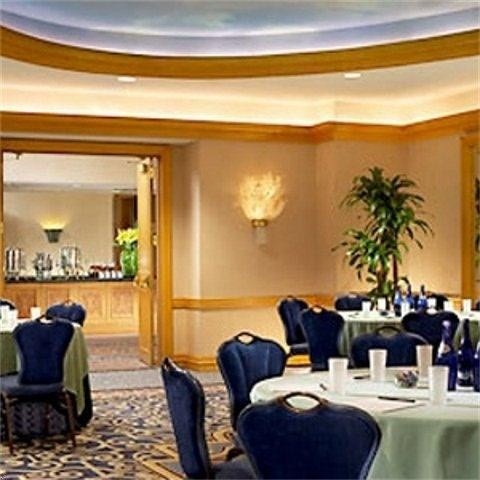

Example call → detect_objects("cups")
325 357 349 394
361 296 474 318
0 304 40 328
367 348 389 382
416 345 434 378
426 365 451 407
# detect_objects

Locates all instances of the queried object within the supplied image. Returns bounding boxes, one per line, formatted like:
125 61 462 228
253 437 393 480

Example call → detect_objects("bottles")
393 284 428 316
474 341 480 392
88 265 124 279
457 317 474 385
435 319 458 394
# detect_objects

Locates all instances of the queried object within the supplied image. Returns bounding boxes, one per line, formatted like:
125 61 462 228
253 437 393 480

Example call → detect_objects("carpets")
81 335 156 374
0 379 236 478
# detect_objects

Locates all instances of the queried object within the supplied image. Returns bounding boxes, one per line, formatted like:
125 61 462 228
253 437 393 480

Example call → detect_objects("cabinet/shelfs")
3 277 137 336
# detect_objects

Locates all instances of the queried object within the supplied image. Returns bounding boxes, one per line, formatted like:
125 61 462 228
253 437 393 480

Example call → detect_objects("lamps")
44 229 63 243
251 219 268 228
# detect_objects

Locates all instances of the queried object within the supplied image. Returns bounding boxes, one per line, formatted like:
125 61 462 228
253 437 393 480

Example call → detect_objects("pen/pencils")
320 384 327 390
378 397 415 402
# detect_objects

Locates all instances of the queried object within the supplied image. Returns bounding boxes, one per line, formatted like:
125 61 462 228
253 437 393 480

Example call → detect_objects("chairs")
333 288 479 369
298 304 350 373
0 298 94 455
278 295 318 368
237 390 382 479
161 355 258 478
216 330 287 462
